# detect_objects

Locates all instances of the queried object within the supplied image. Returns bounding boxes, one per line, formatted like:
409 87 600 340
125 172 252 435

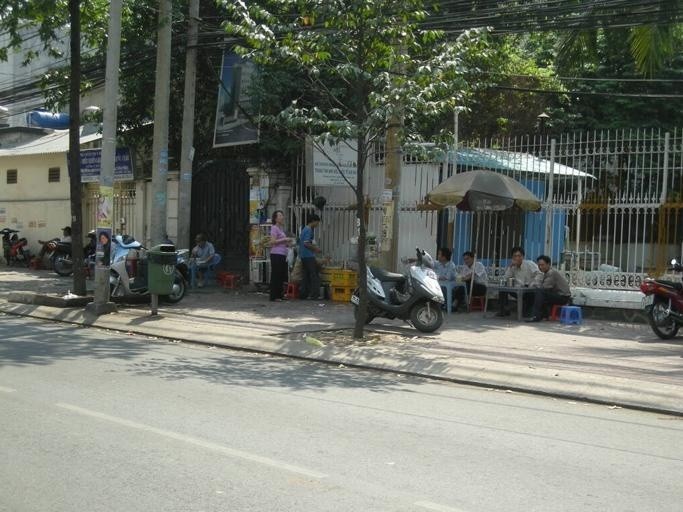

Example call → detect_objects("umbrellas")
425 170 541 304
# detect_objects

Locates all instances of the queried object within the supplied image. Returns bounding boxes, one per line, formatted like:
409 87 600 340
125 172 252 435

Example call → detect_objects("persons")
98 232 110 266
31 227 72 263
434 248 456 310
298 215 323 300
524 255 571 323
451 252 488 312
192 234 215 288
270 210 293 302
496 247 544 316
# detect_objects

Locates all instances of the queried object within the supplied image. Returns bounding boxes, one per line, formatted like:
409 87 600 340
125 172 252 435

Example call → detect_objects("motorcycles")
349 246 445 333
0 227 30 268
108 217 190 304
639 258 682 340
47 217 126 280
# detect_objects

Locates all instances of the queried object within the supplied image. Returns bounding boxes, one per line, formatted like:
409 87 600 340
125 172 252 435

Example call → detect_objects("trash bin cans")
561 251 599 271
147 243 178 315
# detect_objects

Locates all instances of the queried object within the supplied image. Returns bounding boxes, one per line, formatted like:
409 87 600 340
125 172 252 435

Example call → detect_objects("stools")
190 251 302 302
432 276 585 327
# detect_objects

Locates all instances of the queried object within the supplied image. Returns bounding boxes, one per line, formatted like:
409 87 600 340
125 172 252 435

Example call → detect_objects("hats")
61 226 71 231
194 234 206 242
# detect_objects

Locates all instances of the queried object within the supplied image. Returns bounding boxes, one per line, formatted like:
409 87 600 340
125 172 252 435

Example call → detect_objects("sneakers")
524 316 536 322
